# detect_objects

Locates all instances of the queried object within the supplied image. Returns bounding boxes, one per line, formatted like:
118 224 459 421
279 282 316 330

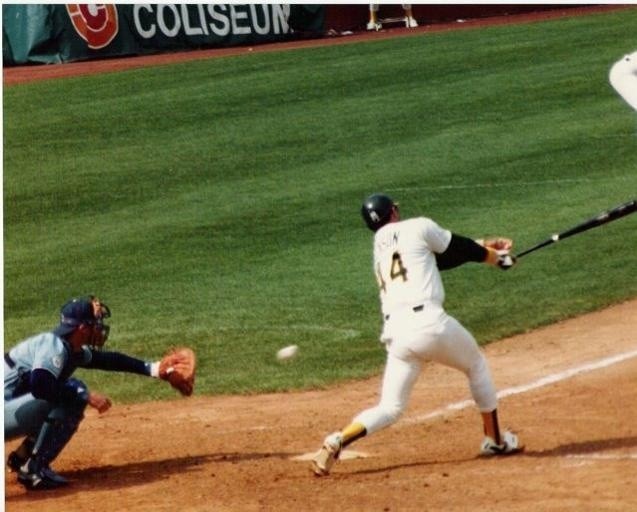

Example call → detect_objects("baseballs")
278 346 299 359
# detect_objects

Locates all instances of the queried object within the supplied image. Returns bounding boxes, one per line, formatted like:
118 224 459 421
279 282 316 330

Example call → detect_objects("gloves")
495 249 517 269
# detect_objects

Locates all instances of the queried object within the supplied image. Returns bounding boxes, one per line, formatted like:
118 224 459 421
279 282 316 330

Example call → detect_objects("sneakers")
481 430 525 456
309 432 343 477
7 451 65 491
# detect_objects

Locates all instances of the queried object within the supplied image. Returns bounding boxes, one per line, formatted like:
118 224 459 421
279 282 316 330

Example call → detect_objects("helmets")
54 295 110 356
361 193 399 234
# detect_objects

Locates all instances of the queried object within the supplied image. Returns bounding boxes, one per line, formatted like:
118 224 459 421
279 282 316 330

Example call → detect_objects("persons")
309 195 525 476
4 294 195 494
368 5 419 30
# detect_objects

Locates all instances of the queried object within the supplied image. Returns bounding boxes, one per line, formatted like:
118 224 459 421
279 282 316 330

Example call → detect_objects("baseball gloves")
160 348 197 397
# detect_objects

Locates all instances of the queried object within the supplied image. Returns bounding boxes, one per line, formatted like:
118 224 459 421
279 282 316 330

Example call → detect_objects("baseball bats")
505 198 636 268
